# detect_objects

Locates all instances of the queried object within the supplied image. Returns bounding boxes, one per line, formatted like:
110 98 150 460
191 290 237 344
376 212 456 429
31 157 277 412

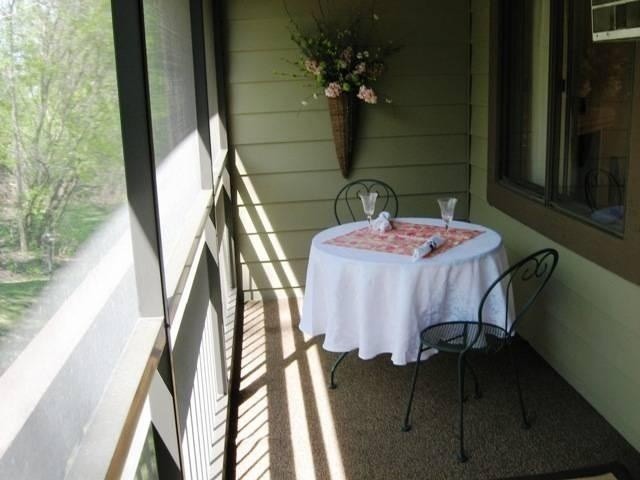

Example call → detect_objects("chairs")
400 248 558 461
333 178 399 225
584 168 622 214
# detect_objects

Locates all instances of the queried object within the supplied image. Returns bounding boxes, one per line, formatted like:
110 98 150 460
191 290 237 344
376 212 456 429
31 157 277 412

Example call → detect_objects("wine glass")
435 197 459 235
357 190 379 230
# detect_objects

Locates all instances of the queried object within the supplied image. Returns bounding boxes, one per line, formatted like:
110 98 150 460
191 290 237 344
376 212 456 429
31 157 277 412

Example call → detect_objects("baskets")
328 91 357 179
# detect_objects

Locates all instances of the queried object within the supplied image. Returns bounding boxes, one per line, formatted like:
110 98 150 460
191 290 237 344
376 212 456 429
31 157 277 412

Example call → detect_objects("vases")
328 95 361 178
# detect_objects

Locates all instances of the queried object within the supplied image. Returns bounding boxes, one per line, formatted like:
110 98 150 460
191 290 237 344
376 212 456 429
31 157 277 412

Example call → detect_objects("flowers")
273 0 406 115
